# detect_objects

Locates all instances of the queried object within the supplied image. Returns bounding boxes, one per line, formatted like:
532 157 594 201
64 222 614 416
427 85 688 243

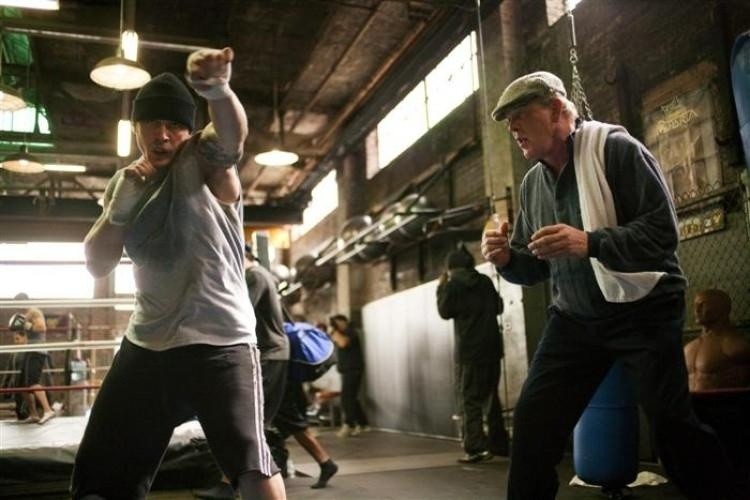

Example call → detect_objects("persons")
10 294 55 426
68 45 287 500
57 313 90 417
193 245 339 500
436 250 510 465
0 328 30 420
482 71 691 500
303 315 363 437
683 288 750 392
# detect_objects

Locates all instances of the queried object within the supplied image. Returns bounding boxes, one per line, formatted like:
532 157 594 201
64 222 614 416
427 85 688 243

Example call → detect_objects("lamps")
89 1 154 93
1 47 47 177
253 79 301 169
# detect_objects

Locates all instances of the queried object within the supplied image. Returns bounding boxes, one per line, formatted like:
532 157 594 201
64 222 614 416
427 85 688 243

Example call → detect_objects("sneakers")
337 424 372 436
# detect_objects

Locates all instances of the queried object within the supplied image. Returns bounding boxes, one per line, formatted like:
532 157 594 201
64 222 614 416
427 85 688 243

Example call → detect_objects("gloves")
10 313 32 332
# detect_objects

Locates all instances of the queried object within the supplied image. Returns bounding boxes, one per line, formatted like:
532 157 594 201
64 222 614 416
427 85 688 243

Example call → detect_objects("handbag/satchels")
282 322 338 381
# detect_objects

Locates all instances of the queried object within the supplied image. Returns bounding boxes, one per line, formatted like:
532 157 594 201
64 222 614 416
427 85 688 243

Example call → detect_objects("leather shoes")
458 451 493 464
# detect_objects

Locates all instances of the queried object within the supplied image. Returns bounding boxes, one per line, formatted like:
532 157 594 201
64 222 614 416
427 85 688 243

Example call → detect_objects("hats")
131 73 197 134
448 248 476 270
492 71 567 121
